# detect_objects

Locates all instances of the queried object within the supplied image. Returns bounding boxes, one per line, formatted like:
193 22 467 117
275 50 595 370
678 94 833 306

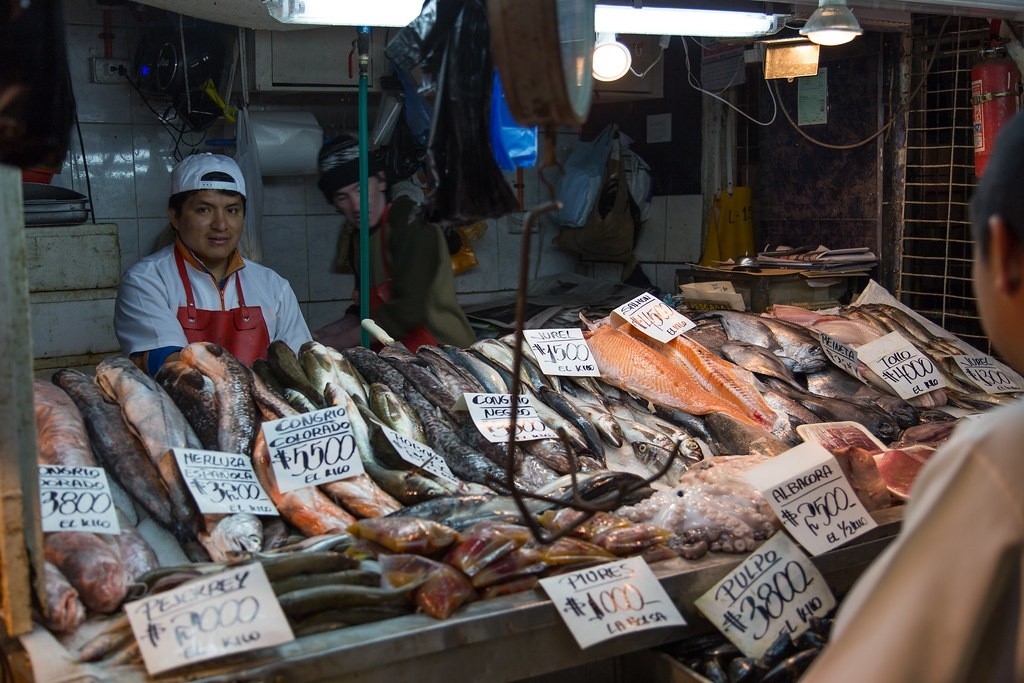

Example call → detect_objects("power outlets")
89 57 133 85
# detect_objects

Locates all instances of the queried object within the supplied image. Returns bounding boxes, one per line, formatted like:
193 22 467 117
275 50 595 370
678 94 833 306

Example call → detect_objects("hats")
171 152 248 196
316 135 388 191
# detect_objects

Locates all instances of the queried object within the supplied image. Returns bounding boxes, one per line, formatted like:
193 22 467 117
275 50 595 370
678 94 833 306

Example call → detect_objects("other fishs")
33 305 1024 680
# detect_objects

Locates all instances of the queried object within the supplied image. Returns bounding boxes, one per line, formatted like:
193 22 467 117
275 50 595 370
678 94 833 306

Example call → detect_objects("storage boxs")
22 222 129 386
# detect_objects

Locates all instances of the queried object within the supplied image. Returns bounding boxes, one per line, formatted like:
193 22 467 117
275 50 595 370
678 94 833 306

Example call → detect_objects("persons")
314 133 479 355
113 150 314 379
790 105 1024 683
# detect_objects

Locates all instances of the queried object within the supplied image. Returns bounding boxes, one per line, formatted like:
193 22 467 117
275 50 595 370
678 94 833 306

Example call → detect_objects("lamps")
591 32 633 83
799 0 863 47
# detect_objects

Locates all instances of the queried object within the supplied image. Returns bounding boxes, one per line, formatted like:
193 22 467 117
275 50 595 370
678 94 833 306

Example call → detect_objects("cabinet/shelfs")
254 24 386 93
